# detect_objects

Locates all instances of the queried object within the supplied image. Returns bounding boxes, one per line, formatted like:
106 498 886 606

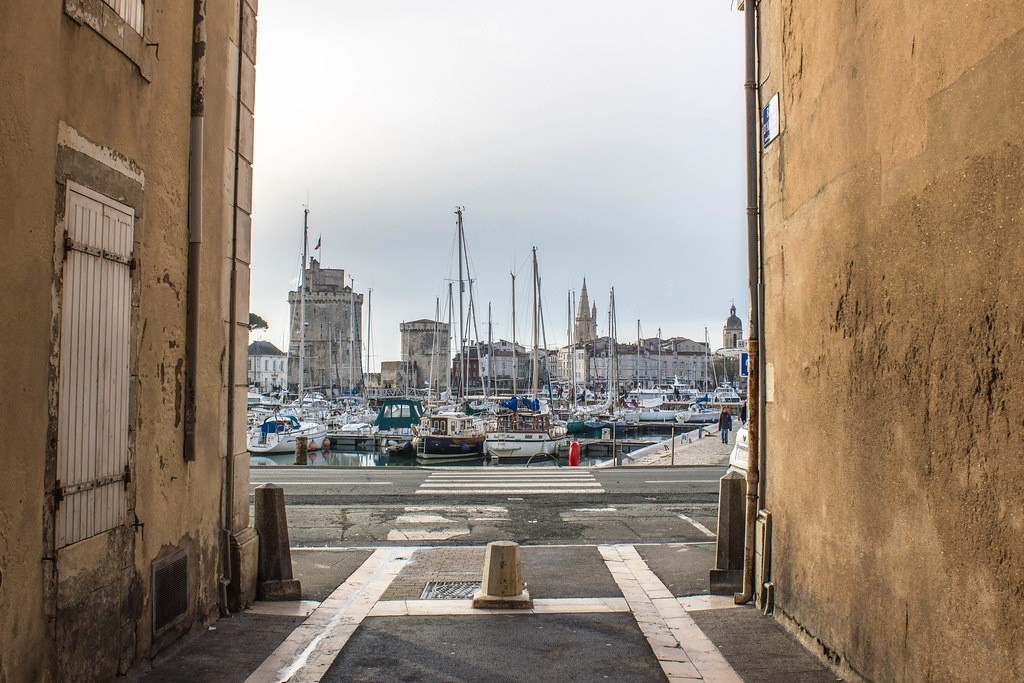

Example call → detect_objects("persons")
740 401 746 425
718 407 732 445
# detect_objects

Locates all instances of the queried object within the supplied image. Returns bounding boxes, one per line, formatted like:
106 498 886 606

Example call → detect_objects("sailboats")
250 194 743 463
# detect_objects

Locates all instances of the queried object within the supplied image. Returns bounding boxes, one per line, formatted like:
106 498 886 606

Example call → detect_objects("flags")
313 238 320 250
